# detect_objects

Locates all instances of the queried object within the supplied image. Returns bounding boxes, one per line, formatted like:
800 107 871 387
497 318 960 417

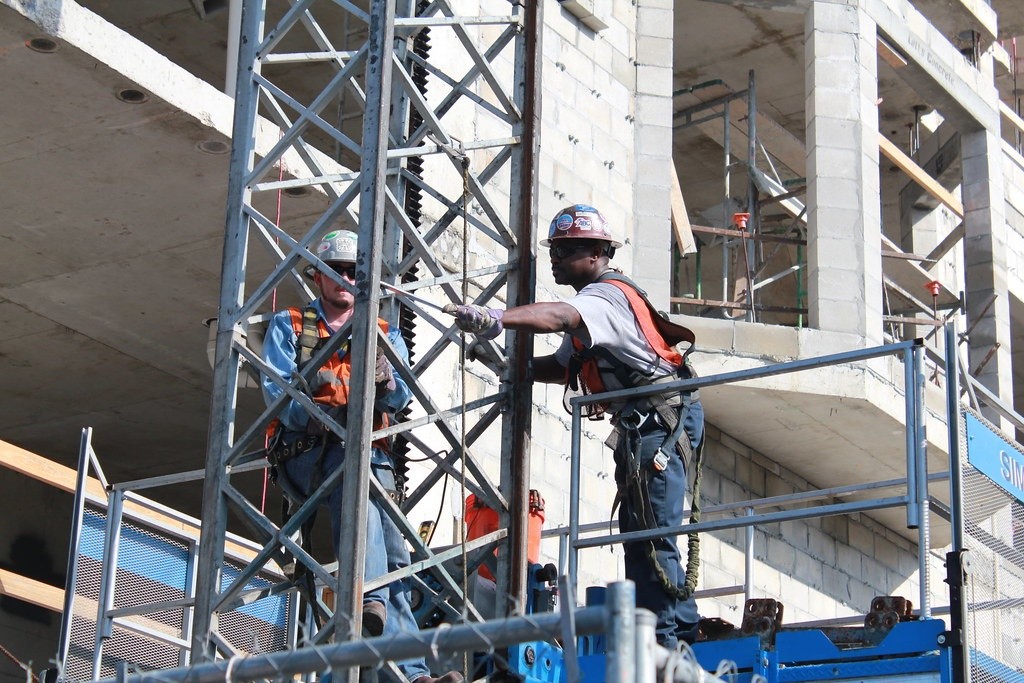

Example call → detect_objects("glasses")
328 264 356 279
549 241 596 259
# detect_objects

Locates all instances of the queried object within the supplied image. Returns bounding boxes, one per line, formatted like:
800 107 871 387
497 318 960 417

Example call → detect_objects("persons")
447 204 705 679
256 228 440 683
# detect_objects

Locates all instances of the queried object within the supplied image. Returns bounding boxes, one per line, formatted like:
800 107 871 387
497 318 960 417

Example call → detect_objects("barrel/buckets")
465 485 546 592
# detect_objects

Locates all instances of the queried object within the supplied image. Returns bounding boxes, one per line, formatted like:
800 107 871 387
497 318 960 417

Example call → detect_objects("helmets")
540 204 624 249
302 230 358 279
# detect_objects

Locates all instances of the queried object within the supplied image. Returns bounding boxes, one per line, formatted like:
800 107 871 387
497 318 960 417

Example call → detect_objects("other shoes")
362 600 387 639
416 669 464 683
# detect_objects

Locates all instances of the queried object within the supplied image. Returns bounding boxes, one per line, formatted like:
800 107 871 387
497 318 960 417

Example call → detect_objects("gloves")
466 338 505 363
442 302 503 340
